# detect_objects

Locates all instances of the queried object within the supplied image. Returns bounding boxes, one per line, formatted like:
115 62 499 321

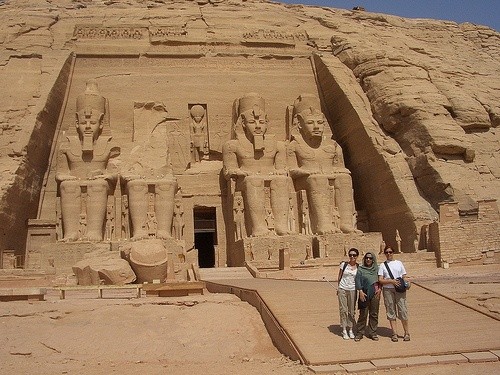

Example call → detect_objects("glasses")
365 257 373 260
385 251 393 254
349 254 357 257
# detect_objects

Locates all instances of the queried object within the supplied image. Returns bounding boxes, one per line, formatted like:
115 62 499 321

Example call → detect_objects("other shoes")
370 334 379 341
354 334 363 341
348 329 355 339
391 334 398 342
403 333 410 341
341 330 349 339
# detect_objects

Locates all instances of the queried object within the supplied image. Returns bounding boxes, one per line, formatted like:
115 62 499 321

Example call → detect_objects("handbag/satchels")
394 277 407 293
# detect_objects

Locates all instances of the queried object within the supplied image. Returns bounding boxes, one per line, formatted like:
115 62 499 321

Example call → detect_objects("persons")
378 245 410 342
354 251 382 341
128 178 177 240
55 94 118 241
224 95 296 237
286 93 363 233
190 105 206 162
337 248 359 341
